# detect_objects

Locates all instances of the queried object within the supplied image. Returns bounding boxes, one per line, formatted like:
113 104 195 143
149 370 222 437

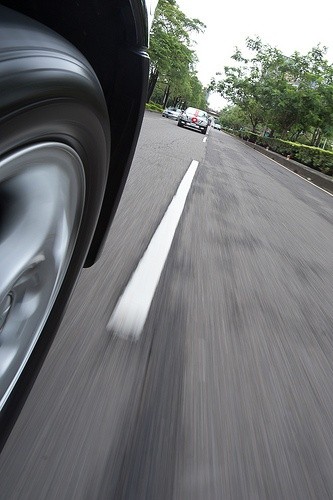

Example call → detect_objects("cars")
208 116 221 131
162 106 182 121
178 107 209 135
